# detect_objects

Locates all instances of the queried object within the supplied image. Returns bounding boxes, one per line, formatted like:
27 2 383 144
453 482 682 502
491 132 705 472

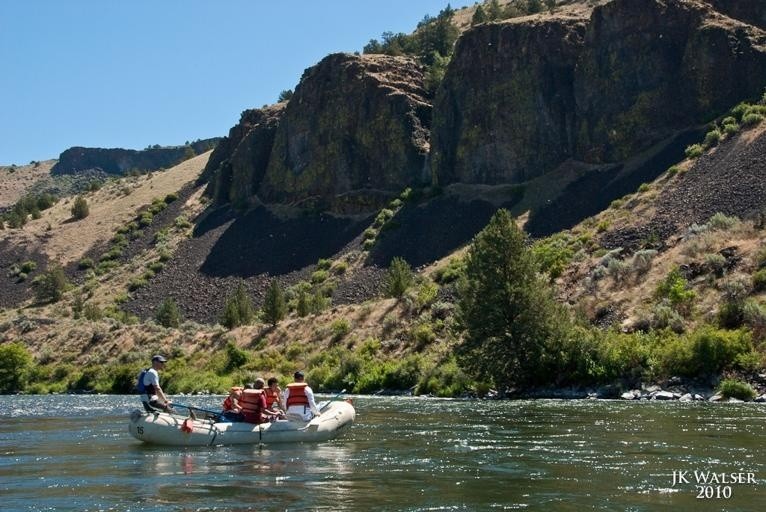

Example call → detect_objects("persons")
136 354 174 413
282 370 321 421
239 378 270 425
222 386 246 422
263 377 286 419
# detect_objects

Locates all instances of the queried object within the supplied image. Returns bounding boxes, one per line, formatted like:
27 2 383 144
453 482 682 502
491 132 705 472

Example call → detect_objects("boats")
126 397 355 445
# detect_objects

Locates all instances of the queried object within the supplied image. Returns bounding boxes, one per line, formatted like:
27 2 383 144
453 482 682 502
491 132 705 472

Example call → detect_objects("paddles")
169 403 244 422
297 389 346 431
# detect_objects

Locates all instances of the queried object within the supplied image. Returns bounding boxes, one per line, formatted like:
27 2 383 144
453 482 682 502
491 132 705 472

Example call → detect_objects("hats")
294 371 304 380
151 355 168 365
243 377 265 389
